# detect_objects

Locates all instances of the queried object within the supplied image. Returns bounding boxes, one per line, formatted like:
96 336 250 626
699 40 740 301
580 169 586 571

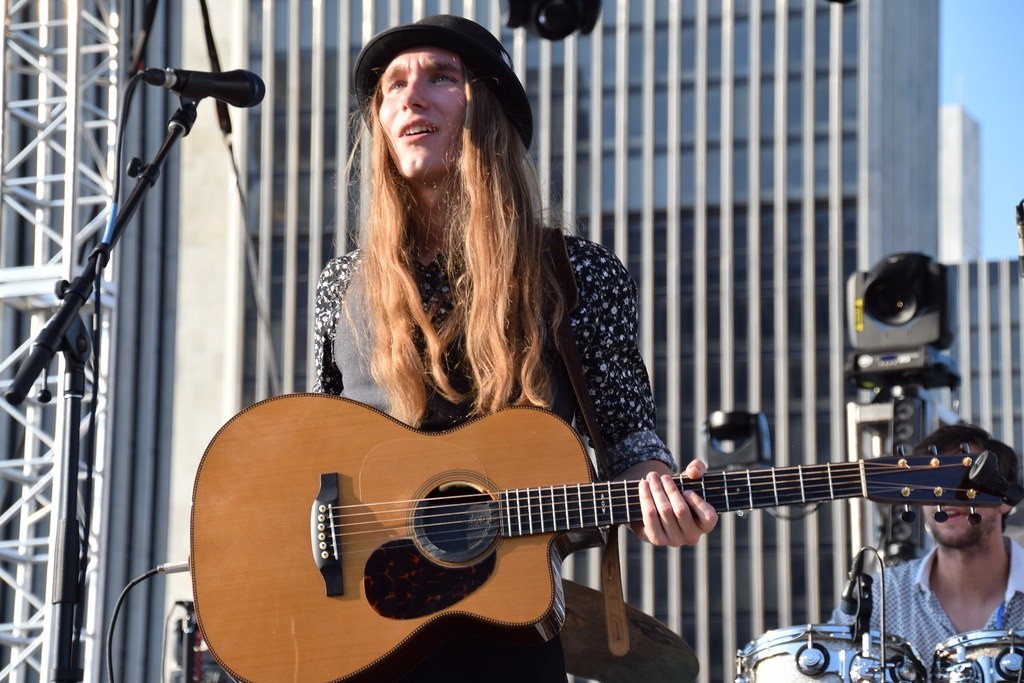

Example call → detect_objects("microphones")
140 67 265 108
840 551 865 615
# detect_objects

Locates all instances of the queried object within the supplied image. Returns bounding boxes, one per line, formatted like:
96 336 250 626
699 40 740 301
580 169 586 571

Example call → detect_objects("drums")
930 632 1024 683
732 624 928 683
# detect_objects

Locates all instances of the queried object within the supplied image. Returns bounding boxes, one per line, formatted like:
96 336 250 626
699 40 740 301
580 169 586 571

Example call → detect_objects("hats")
352 15 533 166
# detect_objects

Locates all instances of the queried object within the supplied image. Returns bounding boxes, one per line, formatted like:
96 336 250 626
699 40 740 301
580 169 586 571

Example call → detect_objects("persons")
311 13 723 682
822 423 1023 683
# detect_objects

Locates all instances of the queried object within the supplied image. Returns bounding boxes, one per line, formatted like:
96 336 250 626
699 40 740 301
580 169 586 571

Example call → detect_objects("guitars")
187 391 1018 683
